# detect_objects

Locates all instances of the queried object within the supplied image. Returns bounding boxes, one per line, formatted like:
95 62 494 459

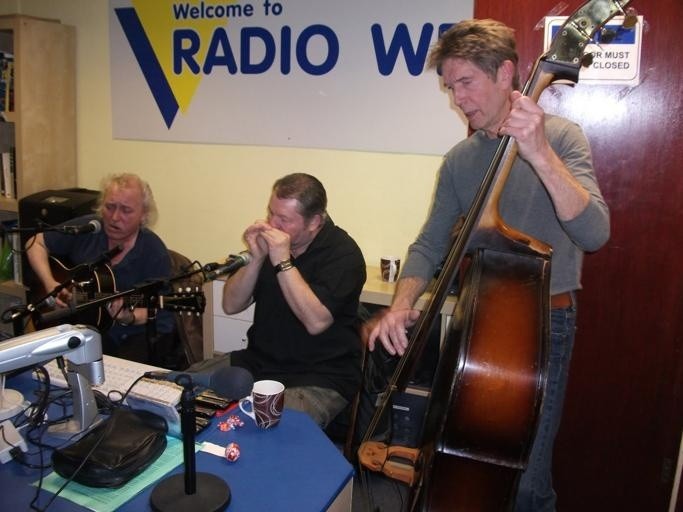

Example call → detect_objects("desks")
0 357 354 511
358 265 459 435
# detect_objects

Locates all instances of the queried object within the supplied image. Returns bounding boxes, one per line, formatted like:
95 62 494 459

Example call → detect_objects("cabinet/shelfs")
0 14 78 337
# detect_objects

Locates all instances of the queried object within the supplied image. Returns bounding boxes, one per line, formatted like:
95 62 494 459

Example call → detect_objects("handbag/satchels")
51 405 169 492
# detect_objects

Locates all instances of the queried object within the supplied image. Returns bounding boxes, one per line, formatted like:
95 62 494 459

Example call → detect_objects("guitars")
25 256 206 353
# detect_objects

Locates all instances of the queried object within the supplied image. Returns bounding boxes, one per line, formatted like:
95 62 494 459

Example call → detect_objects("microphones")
72 218 102 239
143 365 255 402
203 247 253 283
87 241 125 277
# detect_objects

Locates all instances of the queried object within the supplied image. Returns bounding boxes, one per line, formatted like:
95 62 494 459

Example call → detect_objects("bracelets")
119 310 136 327
271 260 294 273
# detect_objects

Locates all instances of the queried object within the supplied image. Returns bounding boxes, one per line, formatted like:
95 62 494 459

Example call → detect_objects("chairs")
168 248 204 374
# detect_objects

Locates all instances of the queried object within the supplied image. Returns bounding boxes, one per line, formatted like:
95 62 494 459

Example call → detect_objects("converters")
0 418 29 465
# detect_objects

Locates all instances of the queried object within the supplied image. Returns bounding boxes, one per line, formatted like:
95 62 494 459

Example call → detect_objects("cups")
240 380 286 430
380 257 400 283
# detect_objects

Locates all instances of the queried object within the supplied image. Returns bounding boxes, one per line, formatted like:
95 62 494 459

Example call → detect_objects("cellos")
351 0 637 512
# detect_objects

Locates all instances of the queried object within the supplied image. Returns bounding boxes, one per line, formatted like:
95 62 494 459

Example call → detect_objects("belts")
548 289 574 311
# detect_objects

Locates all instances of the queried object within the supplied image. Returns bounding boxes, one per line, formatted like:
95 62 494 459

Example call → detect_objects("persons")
25 174 189 373
367 18 614 511
182 174 366 436
